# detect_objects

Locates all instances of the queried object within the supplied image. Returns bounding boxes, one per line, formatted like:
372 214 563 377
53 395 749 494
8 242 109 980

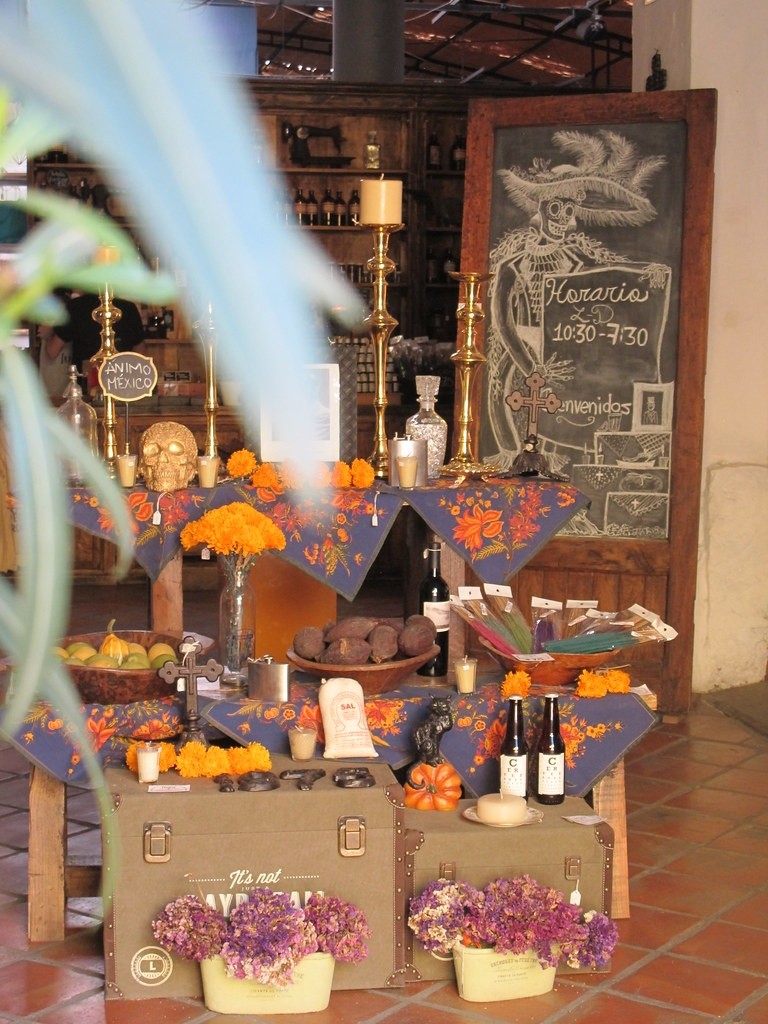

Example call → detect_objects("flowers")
150 887 375 989
226 449 376 490
407 878 620 971
178 499 285 661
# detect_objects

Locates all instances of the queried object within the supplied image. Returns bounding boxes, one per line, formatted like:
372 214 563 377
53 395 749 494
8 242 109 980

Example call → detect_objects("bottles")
416 542 451 676
428 247 458 283
58 366 98 486
330 262 402 283
36 146 94 207
365 129 381 170
293 189 408 226
428 132 466 171
406 376 448 479
500 694 528 802
534 693 565 805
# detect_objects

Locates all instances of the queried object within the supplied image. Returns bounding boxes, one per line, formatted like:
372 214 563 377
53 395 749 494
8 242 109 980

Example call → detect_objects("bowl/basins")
477 634 630 688
286 645 441 695
63 630 185 705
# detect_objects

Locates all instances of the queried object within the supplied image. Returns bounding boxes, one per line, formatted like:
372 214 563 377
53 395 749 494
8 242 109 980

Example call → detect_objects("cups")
453 658 478 696
135 743 162 785
287 720 319 763
394 458 418 491
117 454 138 487
197 456 220 488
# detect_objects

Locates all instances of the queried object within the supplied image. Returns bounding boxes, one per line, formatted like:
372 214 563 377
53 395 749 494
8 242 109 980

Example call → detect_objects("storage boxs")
96 765 402 999
401 796 618 980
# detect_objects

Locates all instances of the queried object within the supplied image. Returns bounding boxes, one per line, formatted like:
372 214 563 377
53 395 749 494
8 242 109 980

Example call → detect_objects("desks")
29 661 658 944
4 468 589 654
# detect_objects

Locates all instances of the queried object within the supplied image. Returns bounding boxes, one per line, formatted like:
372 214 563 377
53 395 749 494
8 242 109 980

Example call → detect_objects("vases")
451 943 555 1003
199 949 336 1014
218 549 259 673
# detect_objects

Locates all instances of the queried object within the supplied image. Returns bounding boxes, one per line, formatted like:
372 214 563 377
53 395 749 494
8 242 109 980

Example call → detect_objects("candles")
359 172 403 226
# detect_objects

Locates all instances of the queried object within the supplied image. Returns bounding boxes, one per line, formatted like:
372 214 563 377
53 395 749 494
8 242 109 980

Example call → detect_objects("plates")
463 806 544 828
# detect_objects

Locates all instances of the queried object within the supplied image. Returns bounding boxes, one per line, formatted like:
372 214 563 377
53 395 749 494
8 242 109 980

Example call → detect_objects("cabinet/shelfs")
15 75 476 394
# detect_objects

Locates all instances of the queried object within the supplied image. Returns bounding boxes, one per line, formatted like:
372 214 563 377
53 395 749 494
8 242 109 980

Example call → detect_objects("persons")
38 293 147 396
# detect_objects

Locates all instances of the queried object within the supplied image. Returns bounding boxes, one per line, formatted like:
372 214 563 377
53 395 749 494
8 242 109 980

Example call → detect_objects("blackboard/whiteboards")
452 88 718 573
98 352 158 401
